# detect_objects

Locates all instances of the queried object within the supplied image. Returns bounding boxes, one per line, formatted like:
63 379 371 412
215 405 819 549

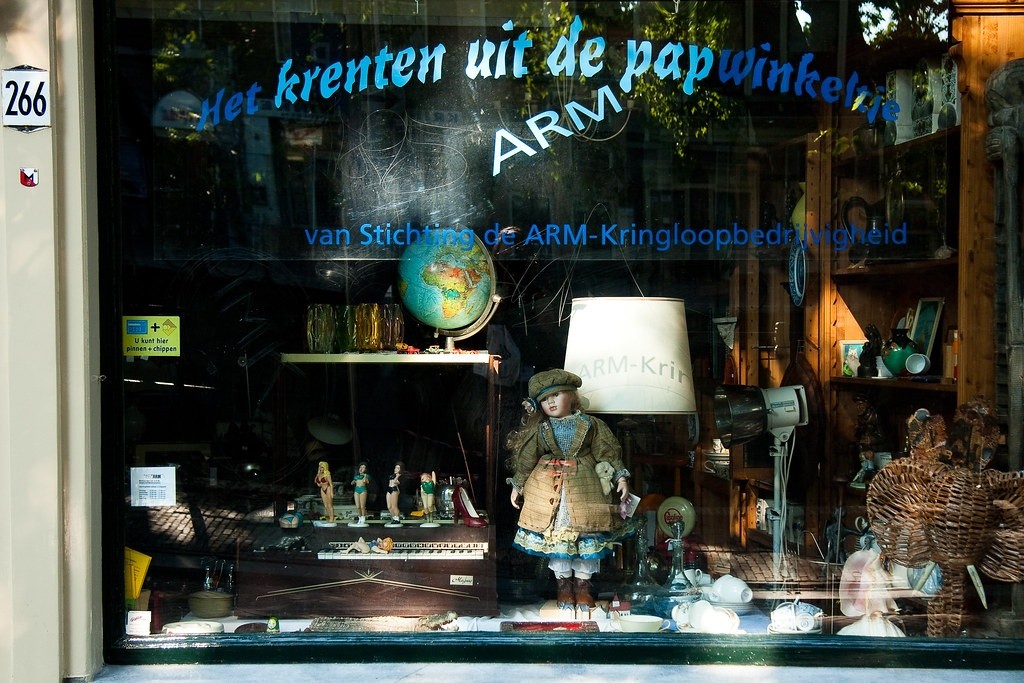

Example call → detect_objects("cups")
310 304 406 352
767 602 824 633
617 567 754 632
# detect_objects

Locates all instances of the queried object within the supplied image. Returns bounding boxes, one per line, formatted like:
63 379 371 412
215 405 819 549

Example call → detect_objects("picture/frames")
908 296 945 359
838 339 869 376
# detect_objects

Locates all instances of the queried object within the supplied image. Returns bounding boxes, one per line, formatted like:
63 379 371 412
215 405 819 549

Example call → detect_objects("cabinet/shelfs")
819 0 1024 563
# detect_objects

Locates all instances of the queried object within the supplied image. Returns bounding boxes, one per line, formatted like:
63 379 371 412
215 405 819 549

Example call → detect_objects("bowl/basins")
875 452 893 470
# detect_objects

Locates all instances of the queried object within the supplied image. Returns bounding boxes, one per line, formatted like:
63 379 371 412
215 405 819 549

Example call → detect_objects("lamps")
715 381 809 582
562 296 697 583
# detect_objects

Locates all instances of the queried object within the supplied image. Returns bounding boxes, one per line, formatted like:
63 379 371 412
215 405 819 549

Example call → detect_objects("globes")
397 221 502 354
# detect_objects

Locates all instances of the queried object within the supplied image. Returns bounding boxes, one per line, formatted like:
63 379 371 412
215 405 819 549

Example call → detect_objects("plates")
788 238 810 305
163 621 225 634
